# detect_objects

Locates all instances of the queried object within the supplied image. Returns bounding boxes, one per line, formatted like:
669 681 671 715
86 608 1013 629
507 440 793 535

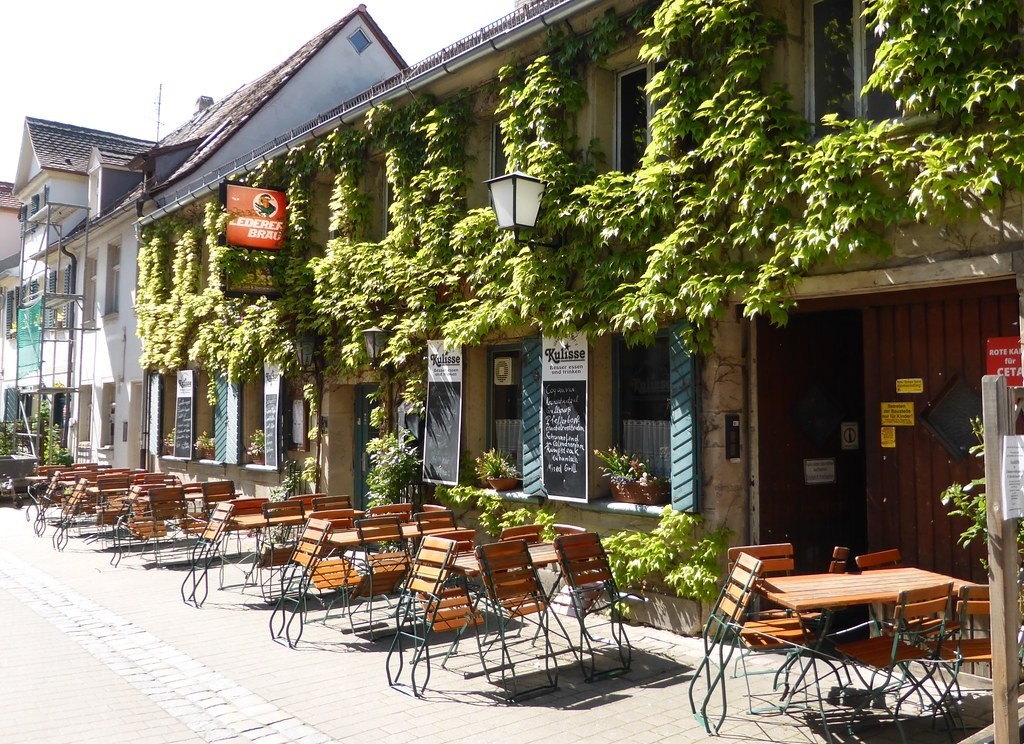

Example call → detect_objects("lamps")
480 161 561 249
295 333 317 374
361 325 393 368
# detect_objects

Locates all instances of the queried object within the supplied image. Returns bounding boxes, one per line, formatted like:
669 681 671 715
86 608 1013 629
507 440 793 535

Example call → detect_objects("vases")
487 477 521 490
607 479 670 505
165 446 265 465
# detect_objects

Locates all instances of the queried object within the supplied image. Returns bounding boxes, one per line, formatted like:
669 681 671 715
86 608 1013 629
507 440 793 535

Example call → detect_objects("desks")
24 475 75 535
751 568 977 744
299 520 469 638
84 484 183 551
442 540 592 683
56 478 182 534
115 492 242 567
218 508 367 594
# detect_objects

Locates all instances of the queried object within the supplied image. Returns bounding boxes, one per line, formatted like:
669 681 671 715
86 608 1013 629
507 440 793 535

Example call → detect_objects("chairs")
26 464 994 744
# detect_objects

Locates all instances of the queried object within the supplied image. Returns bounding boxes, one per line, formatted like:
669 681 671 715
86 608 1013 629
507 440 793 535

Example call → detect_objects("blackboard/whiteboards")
174 396 193 461
421 380 463 486
540 379 589 504
264 392 278 471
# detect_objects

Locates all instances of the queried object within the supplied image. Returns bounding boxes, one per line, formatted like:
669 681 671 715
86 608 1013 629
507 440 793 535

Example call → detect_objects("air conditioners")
494 357 520 385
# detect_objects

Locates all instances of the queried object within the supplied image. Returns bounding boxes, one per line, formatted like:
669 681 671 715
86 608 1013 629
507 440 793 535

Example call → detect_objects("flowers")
473 447 522 480
246 428 265 455
163 427 215 450
593 444 670 488
